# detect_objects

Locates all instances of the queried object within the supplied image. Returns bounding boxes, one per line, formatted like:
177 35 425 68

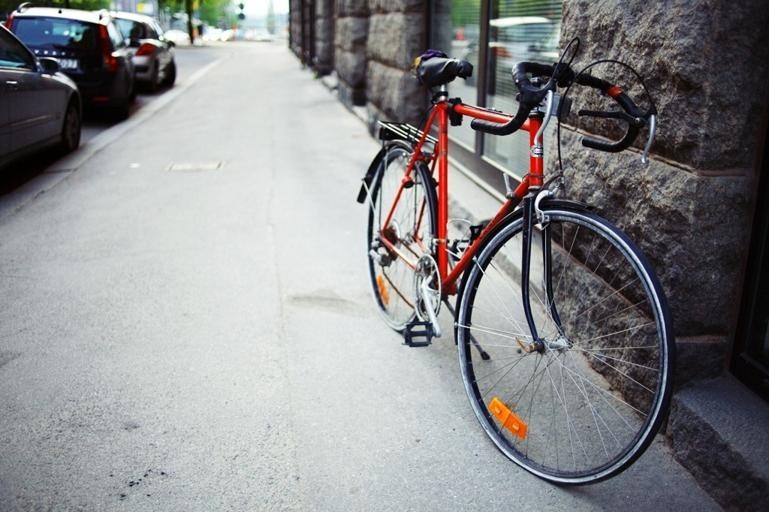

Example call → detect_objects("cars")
449 17 564 97
0 0 189 171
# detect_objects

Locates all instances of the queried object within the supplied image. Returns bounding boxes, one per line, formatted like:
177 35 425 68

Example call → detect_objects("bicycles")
356 36 679 487
